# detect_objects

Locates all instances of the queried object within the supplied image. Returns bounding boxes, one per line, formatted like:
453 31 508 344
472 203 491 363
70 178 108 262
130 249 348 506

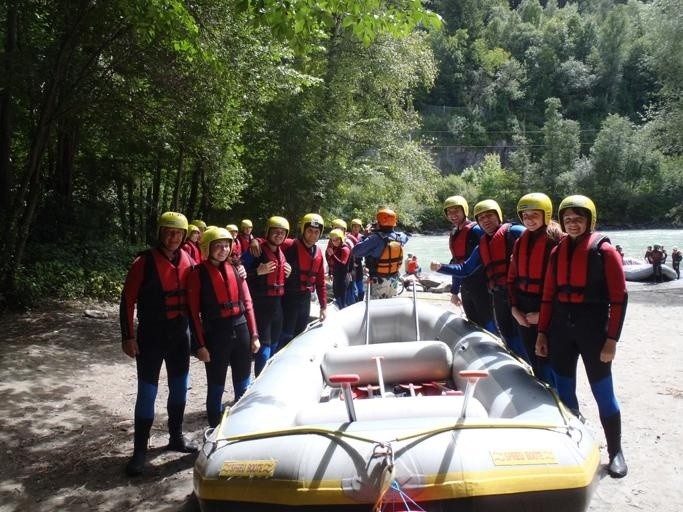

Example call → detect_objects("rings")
534 350 537 352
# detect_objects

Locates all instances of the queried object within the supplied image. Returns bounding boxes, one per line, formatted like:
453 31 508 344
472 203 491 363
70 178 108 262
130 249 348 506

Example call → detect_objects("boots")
166 402 198 453
125 418 153 476
600 410 627 479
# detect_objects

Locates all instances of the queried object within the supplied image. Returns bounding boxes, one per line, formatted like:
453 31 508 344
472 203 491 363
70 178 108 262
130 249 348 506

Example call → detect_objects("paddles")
400 381 422 397
357 384 380 399
423 380 464 396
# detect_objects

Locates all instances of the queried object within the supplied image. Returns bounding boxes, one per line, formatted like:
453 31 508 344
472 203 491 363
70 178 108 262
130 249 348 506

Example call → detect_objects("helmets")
442 195 468 217
376 208 396 227
158 211 188 243
264 216 289 242
199 228 233 257
329 219 361 243
300 213 324 239
473 199 501 223
240 219 252 233
558 196 598 235
187 219 238 238
515 193 553 227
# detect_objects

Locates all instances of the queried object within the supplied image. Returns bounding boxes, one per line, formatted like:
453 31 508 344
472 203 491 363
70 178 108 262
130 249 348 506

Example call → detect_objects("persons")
185 224 262 428
644 246 654 265
116 209 198 474
181 207 424 379
671 247 683 280
649 244 664 284
615 245 624 263
532 193 633 480
443 192 498 339
427 197 528 366
656 245 668 264
506 191 562 385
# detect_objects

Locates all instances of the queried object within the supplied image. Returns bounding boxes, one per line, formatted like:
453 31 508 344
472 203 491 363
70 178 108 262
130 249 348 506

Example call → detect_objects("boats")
622 258 678 282
195 295 598 507
